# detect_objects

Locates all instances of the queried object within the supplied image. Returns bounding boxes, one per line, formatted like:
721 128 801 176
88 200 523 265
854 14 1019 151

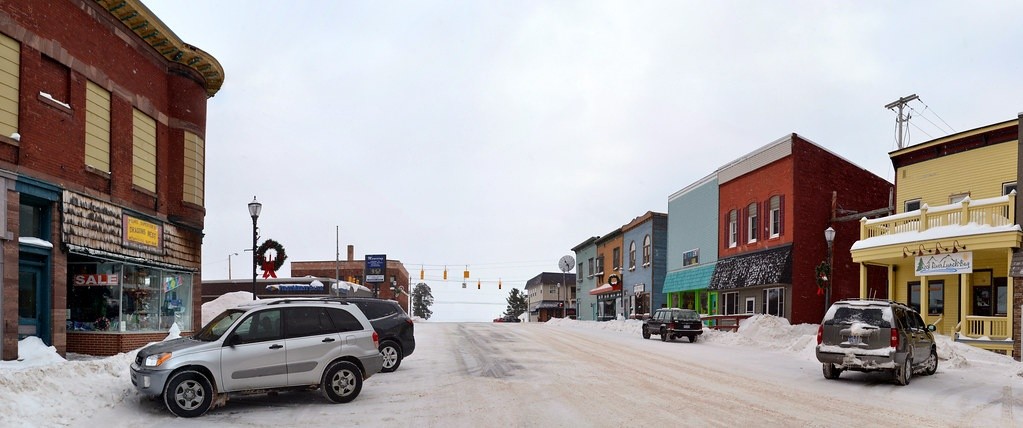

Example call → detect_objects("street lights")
823 226 836 316
248 194 263 300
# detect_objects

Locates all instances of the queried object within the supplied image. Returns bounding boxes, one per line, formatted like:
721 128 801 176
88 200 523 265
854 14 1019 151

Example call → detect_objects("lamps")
902 238 966 259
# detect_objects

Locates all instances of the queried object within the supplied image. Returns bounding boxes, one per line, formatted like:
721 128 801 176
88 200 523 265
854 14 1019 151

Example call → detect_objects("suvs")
642 307 704 343
815 298 939 387
129 298 381 419
238 297 417 374
